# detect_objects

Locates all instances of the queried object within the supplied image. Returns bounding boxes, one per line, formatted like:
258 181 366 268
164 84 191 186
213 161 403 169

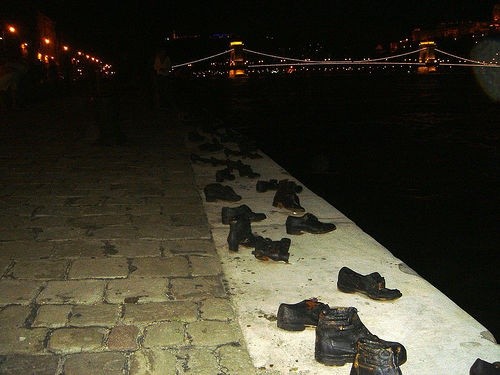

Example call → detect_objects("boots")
350 338 403 375
227 217 258 252
314 306 406 366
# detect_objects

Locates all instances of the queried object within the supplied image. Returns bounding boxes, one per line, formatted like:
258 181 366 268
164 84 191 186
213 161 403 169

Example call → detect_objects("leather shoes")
254 236 292 261
336 265 401 301
285 212 336 235
278 178 303 194
239 164 261 178
254 179 278 193
216 169 235 182
272 188 306 213
205 183 242 204
274 297 329 332
223 203 266 223
